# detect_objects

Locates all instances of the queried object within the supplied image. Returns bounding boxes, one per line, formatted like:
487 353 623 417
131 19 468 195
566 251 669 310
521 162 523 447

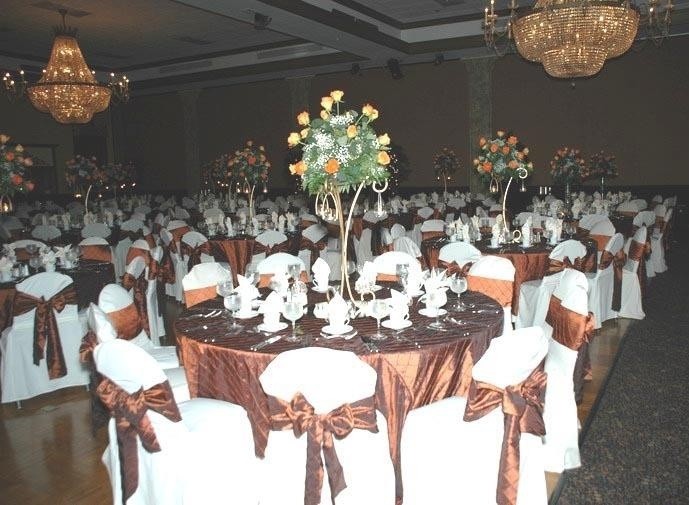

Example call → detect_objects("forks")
177 293 500 355
13 261 110 284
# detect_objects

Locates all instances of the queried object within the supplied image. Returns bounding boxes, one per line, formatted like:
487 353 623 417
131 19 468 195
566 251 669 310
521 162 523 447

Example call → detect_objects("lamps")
482 0 674 80
2 9 131 125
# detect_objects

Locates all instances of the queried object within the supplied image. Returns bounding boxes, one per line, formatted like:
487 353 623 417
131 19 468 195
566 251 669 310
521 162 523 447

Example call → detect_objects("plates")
446 219 594 249
215 260 468 343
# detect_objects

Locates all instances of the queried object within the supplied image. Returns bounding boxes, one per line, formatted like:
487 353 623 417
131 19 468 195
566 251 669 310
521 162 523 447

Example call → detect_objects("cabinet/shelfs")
489 167 529 245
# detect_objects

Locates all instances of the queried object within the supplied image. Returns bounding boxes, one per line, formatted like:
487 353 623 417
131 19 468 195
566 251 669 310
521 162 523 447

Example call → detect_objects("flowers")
201 141 271 194
549 147 590 184
288 90 399 196
1 134 138 195
590 150 619 179
432 146 462 176
472 130 534 183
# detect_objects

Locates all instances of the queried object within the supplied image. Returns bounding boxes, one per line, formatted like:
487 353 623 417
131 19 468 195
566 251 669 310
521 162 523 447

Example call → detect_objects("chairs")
401 325 552 503
92 340 252 505
2 193 678 402
258 348 395 505
533 269 591 476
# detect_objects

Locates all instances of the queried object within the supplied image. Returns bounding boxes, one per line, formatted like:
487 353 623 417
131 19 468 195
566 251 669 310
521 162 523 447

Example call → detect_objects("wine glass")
536 202 596 218
0 244 82 283
